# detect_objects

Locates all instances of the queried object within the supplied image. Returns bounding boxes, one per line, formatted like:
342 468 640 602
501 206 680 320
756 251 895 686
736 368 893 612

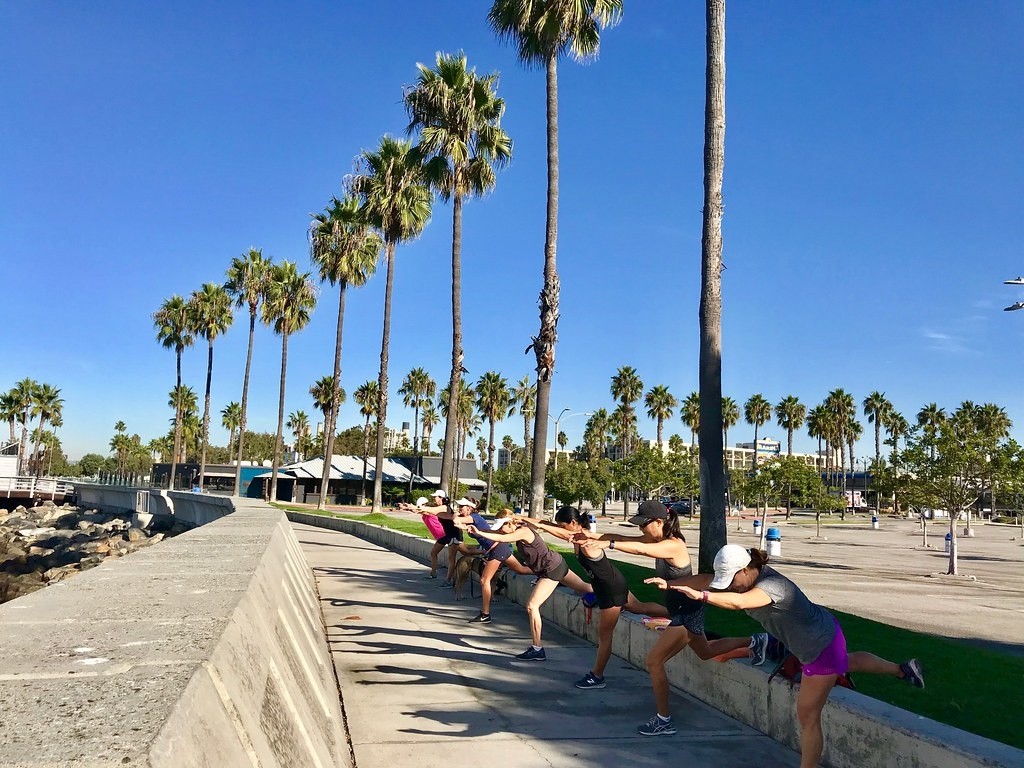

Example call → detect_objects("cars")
651 495 699 515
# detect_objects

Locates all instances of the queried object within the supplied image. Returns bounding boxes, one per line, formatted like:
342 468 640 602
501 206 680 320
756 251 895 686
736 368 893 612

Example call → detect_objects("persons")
513 505 667 690
578 500 753 737
643 544 925 768
396 489 594 662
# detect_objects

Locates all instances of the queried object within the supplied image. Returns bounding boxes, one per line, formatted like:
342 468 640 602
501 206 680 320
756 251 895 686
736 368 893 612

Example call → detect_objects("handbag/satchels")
641 616 673 631
767 652 856 690
581 591 599 609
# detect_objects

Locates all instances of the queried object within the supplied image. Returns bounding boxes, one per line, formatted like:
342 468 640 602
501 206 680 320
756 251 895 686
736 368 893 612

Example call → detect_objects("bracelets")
608 539 615 550
701 591 709 602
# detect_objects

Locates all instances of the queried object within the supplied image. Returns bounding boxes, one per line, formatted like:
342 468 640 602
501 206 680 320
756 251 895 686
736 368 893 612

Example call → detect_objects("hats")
628 500 668 525
489 517 512 531
430 490 446 498
416 496 429 507
709 544 752 589
454 497 476 509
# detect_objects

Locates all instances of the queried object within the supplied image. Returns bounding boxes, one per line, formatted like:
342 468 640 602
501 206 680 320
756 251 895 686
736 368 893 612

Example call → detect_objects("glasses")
639 517 658 529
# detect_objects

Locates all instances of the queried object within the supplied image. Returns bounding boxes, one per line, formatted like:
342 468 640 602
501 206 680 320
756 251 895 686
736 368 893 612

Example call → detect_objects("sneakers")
469 610 492 623
515 644 547 660
438 580 453 588
747 632 769 666
575 671 607 688
424 573 437 579
637 714 678 735
899 658 925 690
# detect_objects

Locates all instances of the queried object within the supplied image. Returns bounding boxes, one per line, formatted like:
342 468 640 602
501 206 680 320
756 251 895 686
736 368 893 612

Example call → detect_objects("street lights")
499 446 525 480
855 456 875 502
519 407 595 522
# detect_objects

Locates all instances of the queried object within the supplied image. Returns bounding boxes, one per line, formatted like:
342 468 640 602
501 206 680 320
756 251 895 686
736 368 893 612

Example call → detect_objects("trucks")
829 490 867 513
